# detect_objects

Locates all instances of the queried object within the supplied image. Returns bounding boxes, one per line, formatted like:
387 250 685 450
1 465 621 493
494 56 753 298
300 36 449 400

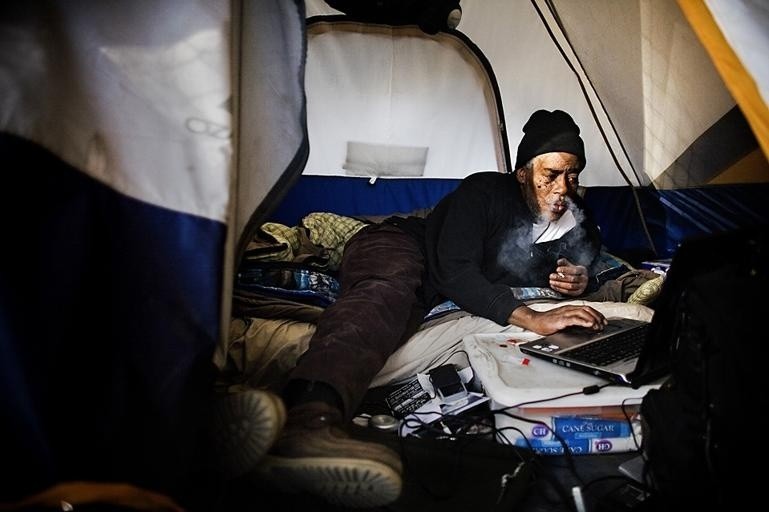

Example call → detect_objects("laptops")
518 230 769 388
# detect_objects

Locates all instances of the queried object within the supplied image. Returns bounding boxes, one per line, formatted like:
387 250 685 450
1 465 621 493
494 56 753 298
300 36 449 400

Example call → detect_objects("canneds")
369 414 398 435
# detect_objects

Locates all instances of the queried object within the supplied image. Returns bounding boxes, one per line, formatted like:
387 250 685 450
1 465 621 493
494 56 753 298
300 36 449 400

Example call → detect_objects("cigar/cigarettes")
558 272 566 279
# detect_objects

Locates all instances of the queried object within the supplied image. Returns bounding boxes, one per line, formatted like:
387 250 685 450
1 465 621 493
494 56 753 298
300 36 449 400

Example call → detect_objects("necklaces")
531 222 550 258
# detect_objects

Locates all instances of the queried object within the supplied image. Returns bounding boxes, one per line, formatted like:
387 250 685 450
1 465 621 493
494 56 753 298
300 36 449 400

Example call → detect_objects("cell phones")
429 364 468 402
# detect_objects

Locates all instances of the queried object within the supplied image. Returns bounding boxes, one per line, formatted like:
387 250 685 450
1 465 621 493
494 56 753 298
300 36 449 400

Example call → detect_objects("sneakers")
205 382 288 478
267 399 405 509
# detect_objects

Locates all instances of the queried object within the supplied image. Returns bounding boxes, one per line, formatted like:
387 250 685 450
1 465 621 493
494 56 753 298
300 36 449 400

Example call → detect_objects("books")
383 376 490 437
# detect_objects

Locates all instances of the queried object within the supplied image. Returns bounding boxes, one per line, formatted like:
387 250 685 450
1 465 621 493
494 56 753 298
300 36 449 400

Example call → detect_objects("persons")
209 109 609 510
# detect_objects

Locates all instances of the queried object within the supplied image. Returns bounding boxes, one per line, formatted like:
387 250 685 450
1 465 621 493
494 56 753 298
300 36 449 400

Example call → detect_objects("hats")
515 109 587 173
